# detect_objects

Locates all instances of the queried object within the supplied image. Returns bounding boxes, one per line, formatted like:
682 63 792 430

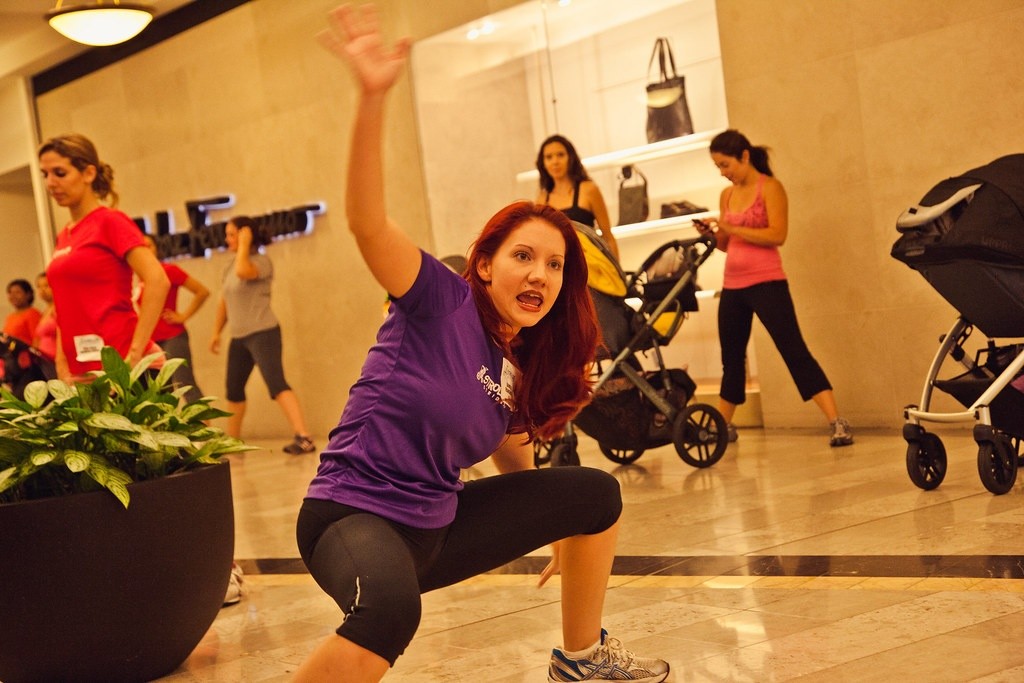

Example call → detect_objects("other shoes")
660 201 709 219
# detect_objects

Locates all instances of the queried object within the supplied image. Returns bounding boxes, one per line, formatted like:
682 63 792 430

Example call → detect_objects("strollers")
890 152 1024 497
533 236 728 469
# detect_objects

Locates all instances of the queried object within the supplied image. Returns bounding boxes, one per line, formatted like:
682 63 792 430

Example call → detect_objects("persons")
209 215 316 455
34 272 57 381
694 129 853 447
136 234 210 428
0 279 42 380
36 133 173 396
538 134 619 259
286 4 671 683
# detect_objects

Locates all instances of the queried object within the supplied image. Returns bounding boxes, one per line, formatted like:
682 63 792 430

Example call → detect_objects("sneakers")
829 417 852 446
284 434 316 454
223 562 244 604
701 423 737 442
546 628 670 683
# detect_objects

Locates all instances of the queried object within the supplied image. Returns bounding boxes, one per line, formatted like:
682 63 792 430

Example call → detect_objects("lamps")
43 0 158 47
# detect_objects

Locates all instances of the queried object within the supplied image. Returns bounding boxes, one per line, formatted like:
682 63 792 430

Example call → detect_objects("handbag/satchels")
645 37 694 143
615 164 648 223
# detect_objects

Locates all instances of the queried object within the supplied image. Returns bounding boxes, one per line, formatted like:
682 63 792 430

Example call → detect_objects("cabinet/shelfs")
517 124 752 378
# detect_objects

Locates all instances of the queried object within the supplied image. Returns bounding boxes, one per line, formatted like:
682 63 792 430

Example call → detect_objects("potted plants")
0 345 276 683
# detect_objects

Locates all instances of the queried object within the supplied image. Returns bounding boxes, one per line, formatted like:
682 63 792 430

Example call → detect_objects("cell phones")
693 219 715 235
